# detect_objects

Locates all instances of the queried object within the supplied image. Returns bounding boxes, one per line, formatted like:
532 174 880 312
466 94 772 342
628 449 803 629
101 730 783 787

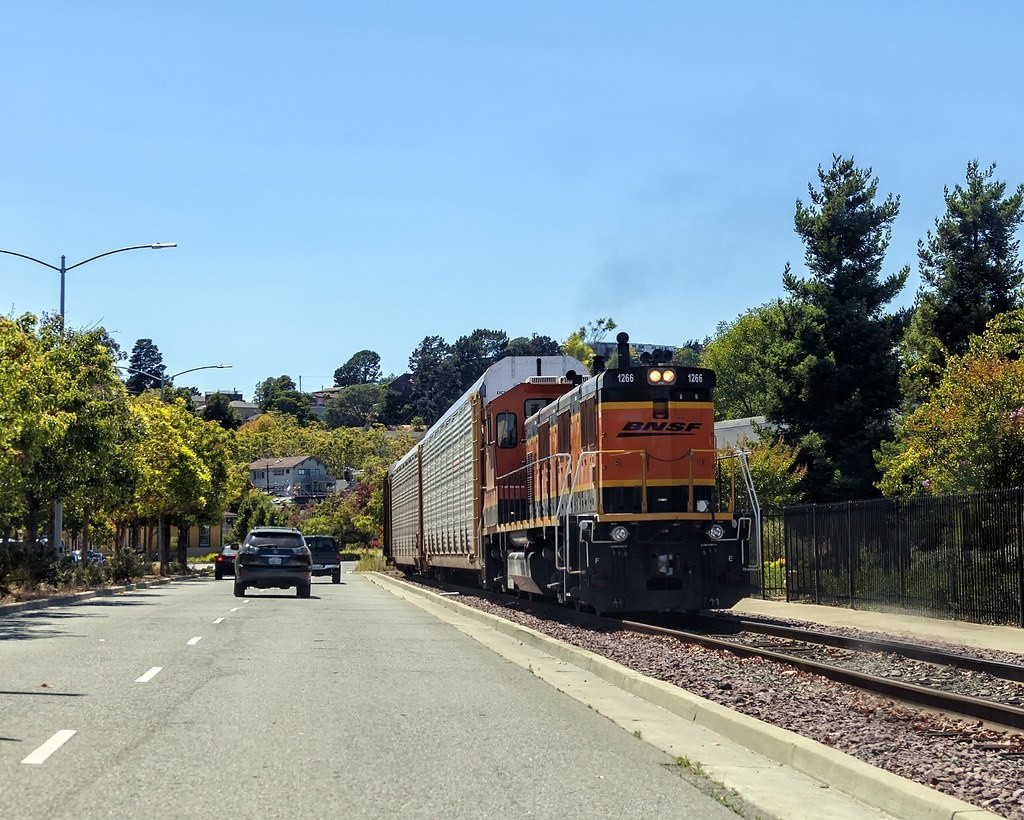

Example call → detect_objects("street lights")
1 243 181 554
112 365 234 569
266 460 281 493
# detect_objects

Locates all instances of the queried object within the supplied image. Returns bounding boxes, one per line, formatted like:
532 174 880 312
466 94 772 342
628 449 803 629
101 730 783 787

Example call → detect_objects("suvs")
303 535 341 582
231 526 317 599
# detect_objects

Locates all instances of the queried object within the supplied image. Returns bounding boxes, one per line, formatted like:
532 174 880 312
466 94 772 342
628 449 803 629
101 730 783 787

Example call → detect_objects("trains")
381 331 762 630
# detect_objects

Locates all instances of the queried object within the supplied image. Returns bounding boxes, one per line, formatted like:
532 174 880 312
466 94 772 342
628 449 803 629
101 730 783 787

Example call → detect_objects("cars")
214 543 240 579
66 550 104 569
